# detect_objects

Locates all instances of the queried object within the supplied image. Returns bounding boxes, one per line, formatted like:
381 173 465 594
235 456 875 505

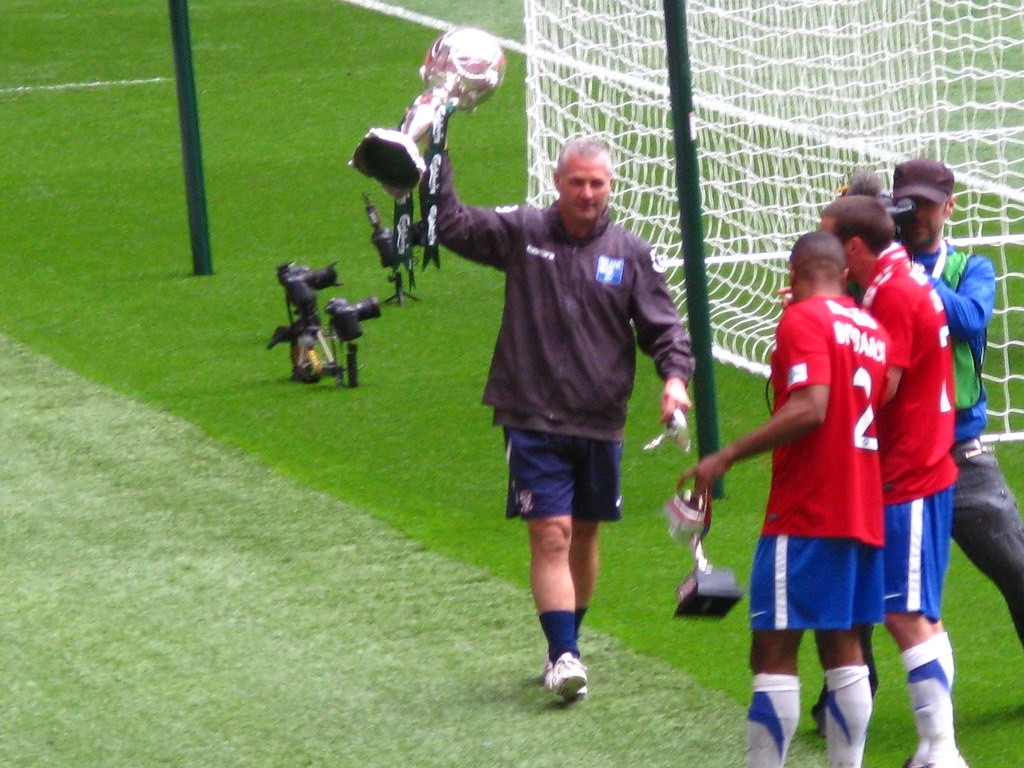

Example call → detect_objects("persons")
817 195 971 768
419 130 696 702
890 159 1024 650
675 231 894 768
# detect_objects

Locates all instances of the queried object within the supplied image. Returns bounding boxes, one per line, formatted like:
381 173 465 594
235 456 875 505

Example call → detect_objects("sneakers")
542 652 589 699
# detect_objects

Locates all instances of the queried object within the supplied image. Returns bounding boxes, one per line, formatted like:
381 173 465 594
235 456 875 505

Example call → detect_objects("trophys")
665 487 746 621
350 24 506 199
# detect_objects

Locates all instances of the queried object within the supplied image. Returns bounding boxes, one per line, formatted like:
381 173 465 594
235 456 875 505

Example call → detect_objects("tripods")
266 307 346 389
385 266 421 309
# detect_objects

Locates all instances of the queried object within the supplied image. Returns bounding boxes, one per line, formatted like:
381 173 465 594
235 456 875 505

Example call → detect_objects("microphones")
845 169 884 197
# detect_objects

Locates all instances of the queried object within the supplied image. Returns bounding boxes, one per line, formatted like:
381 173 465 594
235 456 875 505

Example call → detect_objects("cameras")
372 220 428 267
276 259 344 313
325 296 381 342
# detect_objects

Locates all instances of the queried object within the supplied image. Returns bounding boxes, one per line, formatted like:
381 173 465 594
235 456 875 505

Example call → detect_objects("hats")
894 159 955 203
